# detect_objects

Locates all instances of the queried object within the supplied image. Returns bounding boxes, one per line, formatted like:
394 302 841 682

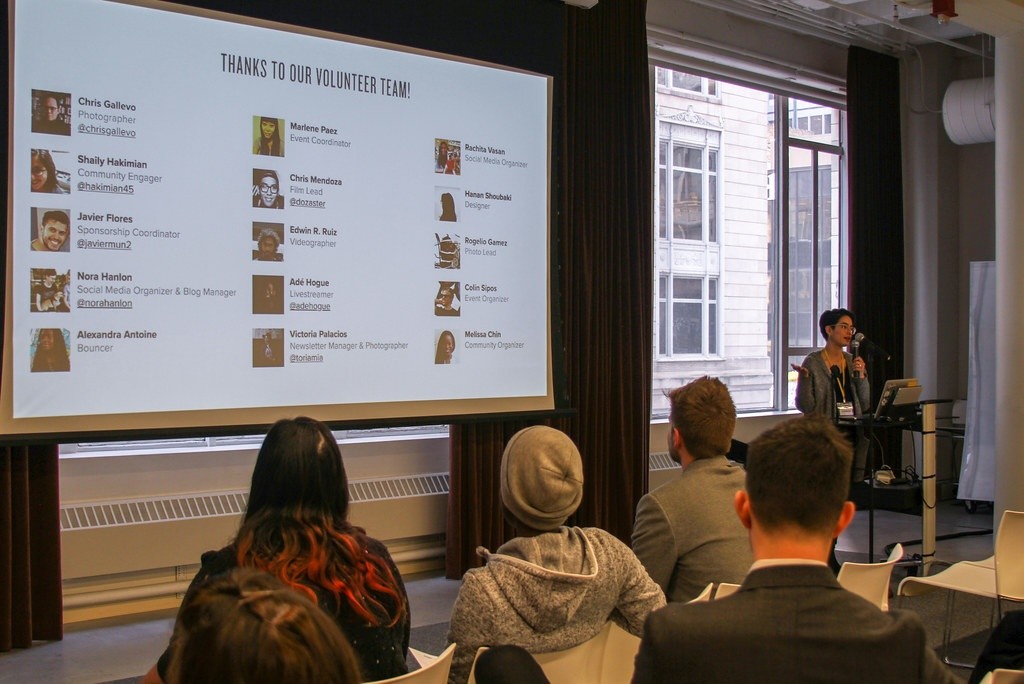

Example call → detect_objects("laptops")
855 378 918 420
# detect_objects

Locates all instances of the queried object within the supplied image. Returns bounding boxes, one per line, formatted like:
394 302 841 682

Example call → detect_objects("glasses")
31 168 47 176
828 324 856 336
42 106 59 111
259 183 279 194
439 294 451 298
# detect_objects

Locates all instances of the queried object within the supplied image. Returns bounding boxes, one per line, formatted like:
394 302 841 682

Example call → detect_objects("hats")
500 425 584 531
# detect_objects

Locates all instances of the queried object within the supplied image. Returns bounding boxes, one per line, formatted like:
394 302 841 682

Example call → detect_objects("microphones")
850 340 860 378
855 333 891 360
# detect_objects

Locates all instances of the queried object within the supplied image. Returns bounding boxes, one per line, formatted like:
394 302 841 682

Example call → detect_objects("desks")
835 417 922 563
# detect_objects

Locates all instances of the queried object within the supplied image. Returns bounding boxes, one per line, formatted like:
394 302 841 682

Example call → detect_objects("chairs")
467 621 612 684
836 543 903 611
897 509 1024 668
363 643 456 684
607 583 713 684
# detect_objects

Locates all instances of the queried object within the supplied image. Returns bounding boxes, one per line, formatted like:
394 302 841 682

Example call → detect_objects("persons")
146 414 411 682
790 309 871 485
30 93 461 371
448 424 668 684
630 414 966 683
634 379 747 600
